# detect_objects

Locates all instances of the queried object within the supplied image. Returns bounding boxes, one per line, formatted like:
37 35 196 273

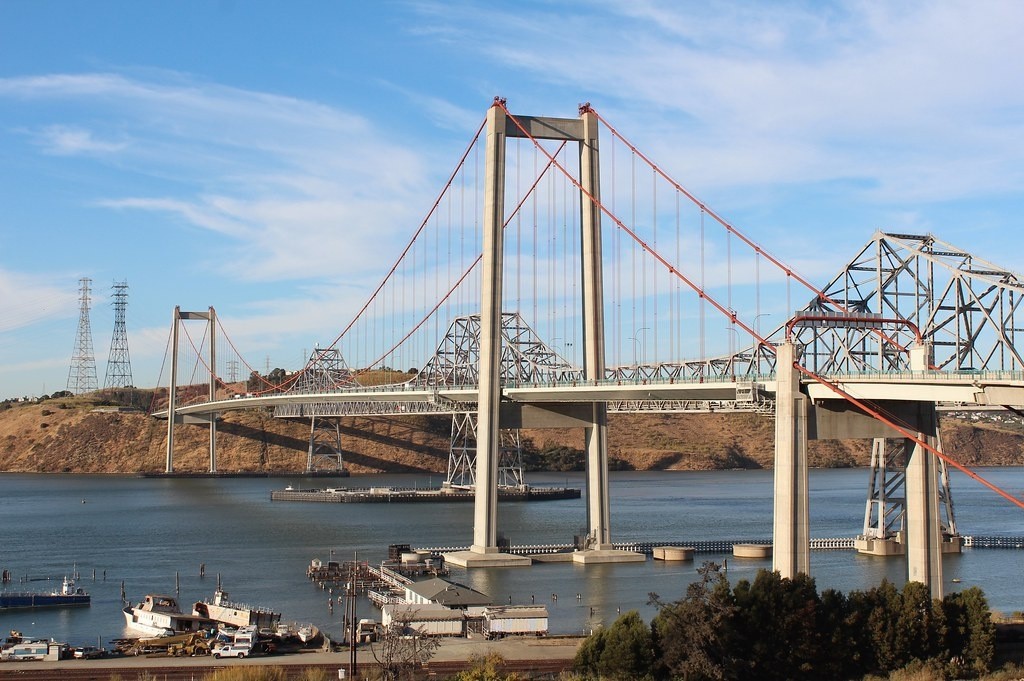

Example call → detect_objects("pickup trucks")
211 645 249 659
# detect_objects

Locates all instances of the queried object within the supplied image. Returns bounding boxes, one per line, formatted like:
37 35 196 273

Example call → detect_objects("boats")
0 575 90 609
297 626 312 642
121 594 208 637
191 590 281 631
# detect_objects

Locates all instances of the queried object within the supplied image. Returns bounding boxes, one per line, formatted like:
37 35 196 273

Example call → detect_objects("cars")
259 627 272 637
277 625 288 634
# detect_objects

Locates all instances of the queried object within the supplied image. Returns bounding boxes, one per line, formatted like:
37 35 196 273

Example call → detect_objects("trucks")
74 648 104 660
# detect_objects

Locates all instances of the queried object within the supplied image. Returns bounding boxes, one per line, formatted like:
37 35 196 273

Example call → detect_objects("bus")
0 644 47 660
234 624 259 650
